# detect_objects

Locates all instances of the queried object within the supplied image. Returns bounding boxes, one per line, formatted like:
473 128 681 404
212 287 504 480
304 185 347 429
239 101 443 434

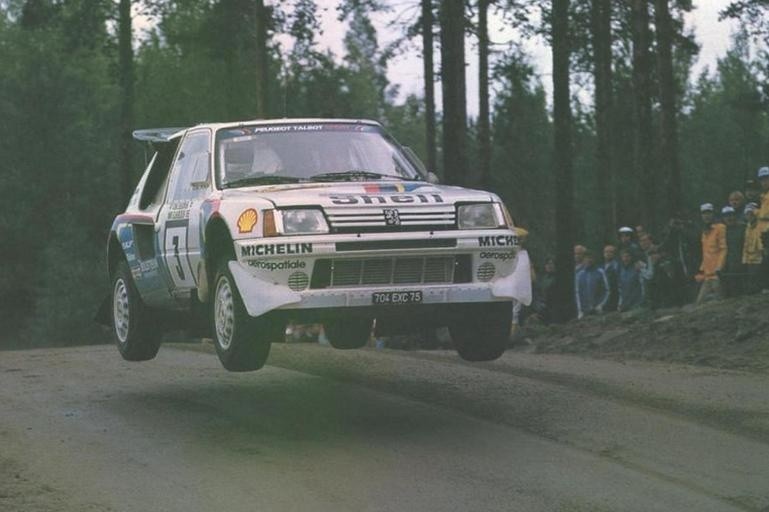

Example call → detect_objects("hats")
700 202 758 213
758 166 769 177
618 226 633 232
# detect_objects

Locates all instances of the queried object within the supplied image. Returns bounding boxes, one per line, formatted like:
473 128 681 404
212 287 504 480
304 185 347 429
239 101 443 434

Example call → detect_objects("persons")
508 168 769 345
284 319 328 344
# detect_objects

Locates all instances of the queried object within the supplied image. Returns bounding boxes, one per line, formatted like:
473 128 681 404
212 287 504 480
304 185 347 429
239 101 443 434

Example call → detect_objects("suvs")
107 116 533 372
